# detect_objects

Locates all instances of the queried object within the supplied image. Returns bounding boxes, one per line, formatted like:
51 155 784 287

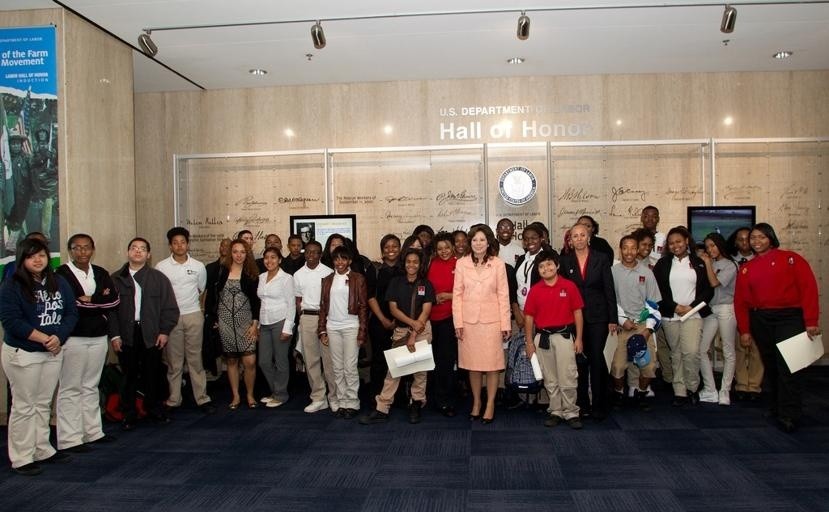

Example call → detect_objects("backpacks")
505 327 543 394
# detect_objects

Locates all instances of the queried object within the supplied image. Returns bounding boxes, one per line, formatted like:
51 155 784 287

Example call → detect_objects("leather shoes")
246 398 259 409
228 400 242 409
147 414 175 426
479 408 496 424
671 389 688 409
733 391 748 407
468 401 484 422
260 396 274 403
265 400 283 408
407 404 424 424
579 409 609 424
686 389 702 409
747 391 762 409
120 411 138 432
504 401 543 413
359 410 389 425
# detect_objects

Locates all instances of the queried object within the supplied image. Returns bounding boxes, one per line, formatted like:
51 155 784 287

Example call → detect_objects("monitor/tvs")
687 206 756 250
290 213 356 255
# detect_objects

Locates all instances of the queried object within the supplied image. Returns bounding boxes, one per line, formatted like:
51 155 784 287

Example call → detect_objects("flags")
12 91 35 157
0 109 14 179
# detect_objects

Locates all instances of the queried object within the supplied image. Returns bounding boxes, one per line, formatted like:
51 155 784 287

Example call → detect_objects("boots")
608 389 625 412
634 389 656 412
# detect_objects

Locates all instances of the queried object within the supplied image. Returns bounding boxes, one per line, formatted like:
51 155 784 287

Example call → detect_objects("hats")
627 334 651 369
8 128 27 142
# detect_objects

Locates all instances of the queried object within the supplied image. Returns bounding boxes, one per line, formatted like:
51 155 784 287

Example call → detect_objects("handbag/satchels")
392 336 409 348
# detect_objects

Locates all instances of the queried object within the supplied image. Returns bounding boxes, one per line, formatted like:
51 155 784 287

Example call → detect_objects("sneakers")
64 443 98 453
220 359 230 372
94 435 117 442
8 460 42 475
698 389 719 403
718 389 732 406
332 408 361 418
303 400 329 413
562 417 584 429
760 405 800 434
160 400 185 412
238 363 245 381
35 452 72 465
439 399 458 417
329 402 339 412
205 370 219 383
543 415 562 427
199 402 218 416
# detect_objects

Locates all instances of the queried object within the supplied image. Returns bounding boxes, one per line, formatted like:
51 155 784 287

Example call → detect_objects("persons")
153 227 216 416
202 229 376 419
361 215 764 429
1 231 121 471
105 237 180 429
0 122 58 257
642 205 669 268
733 223 820 436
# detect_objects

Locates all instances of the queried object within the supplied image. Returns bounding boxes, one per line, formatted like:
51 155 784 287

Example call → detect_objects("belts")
300 309 320 315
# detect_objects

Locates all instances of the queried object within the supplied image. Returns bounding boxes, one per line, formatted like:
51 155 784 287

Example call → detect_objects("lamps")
311 19 327 51
136 30 160 57
719 4 737 33
515 11 530 39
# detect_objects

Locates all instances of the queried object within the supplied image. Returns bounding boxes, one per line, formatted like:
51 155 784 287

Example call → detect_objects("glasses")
70 246 93 251
497 226 513 230
